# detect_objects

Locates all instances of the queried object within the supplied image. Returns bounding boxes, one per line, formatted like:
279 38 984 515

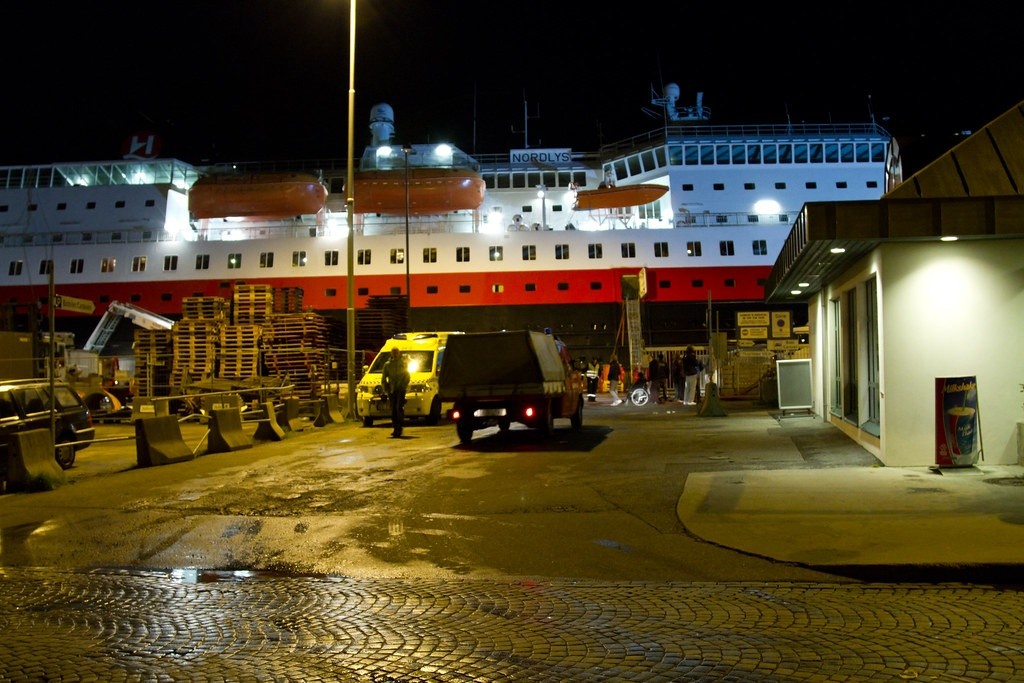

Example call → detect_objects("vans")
354 329 464 427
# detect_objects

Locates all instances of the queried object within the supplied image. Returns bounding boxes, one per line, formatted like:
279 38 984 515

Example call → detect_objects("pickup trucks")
444 330 584 447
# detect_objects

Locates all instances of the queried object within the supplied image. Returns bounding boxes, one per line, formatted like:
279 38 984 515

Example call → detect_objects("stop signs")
742 329 748 336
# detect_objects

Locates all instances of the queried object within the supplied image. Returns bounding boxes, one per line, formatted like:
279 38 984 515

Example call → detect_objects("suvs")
0 379 94 470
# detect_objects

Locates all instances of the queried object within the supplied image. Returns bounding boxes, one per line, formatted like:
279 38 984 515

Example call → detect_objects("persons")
606 353 622 406
672 352 686 401
628 372 646 400
381 348 411 440
582 356 602 402
683 345 702 405
647 355 659 403
656 354 671 400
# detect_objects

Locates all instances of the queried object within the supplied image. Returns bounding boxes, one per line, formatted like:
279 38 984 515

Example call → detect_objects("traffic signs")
55 294 97 315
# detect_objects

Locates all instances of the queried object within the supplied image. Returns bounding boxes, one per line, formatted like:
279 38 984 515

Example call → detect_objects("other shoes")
391 428 403 438
610 400 622 407
688 401 697 405
684 401 688 405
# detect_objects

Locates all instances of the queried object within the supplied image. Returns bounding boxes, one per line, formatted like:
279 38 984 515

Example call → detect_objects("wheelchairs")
629 380 650 407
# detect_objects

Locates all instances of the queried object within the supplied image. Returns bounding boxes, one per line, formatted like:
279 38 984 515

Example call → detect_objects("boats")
344 166 487 215
576 183 670 213
187 160 324 221
1 85 907 384
